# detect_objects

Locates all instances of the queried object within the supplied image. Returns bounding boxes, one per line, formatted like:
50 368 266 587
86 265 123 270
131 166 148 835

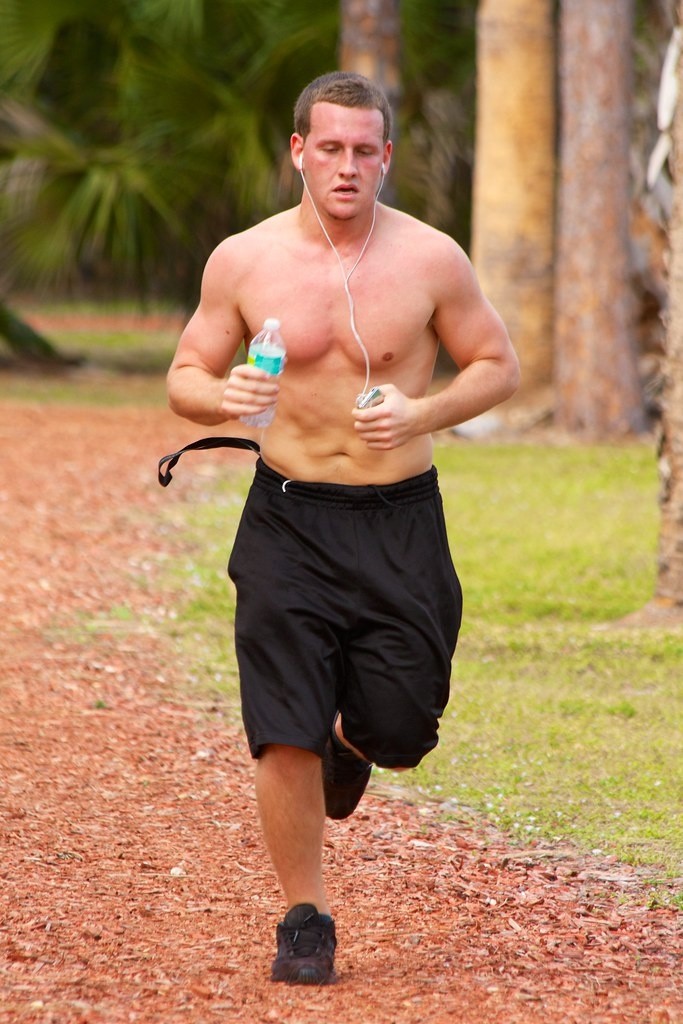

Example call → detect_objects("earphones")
299 151 304 171
381 162 385 176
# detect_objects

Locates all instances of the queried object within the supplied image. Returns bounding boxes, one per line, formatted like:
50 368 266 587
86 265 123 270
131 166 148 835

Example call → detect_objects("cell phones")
358 387 385 409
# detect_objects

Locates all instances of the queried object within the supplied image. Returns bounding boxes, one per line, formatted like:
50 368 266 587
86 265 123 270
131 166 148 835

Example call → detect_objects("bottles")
238 317 286 429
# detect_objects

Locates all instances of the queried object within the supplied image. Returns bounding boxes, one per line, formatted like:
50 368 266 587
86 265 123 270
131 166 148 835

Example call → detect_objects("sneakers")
322 711 373 820
269 903 339 985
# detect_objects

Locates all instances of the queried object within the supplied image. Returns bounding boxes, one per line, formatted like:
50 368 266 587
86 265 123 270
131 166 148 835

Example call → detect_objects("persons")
165 70 519 983
